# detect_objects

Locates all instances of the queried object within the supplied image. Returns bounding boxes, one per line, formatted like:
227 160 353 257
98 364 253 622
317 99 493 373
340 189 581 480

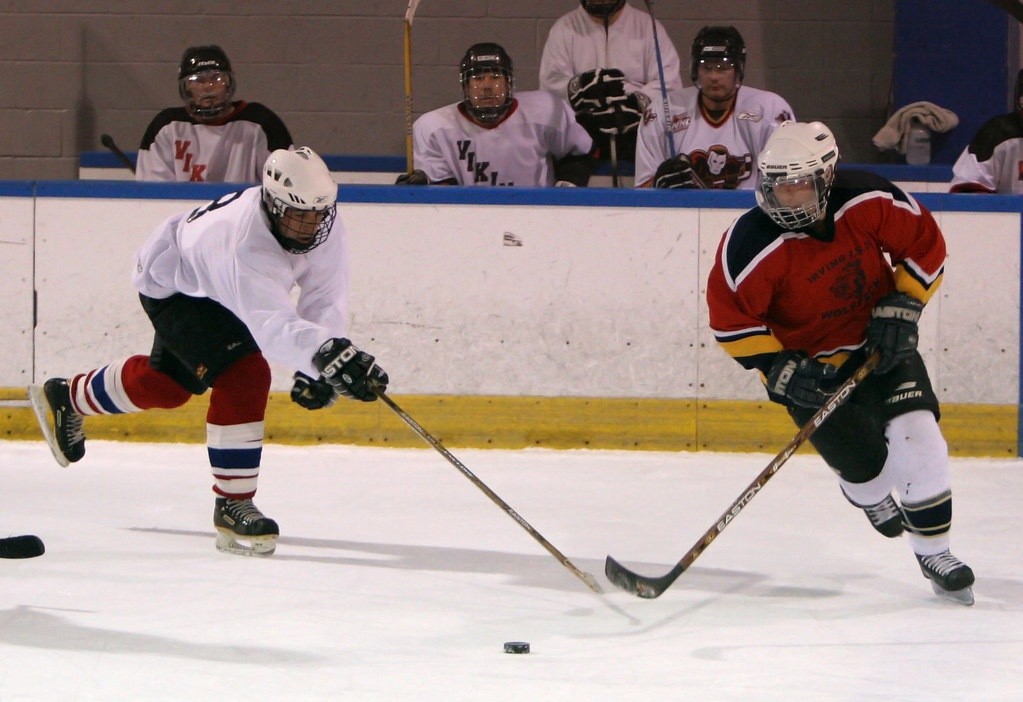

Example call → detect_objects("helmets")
688 23 749 83
458 42 512 90
754 119 840 188
263 143 339 218
176 43 234 79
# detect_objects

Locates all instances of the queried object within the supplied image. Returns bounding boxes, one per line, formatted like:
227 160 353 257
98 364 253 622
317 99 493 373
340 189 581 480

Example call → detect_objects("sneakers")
870 495 906 540
213 494 281 558
916 551 977 609
27 377 88 469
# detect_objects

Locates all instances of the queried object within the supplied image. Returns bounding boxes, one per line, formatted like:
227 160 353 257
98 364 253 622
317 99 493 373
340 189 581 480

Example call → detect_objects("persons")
393 42 602 187
706 120 976 594
633 24 796 190
539 0 683 134
946 68 1023 194
135 45 295 184
44 145 390 542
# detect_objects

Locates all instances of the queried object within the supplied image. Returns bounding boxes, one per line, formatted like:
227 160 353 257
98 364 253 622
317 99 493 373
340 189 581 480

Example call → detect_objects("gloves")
312 337 391 404
290 373 337 412
869 293 926 376
567 67 627 111
762 351 837 410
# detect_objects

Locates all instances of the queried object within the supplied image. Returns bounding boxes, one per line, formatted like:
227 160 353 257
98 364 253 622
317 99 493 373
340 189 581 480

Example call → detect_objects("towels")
872 100 960 156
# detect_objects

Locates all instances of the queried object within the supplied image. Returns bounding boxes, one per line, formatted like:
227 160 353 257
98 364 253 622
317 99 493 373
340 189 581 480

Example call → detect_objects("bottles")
906 123 931 164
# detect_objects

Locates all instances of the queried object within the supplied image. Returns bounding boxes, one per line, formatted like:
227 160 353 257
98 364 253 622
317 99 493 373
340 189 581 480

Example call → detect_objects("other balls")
504 642 530 654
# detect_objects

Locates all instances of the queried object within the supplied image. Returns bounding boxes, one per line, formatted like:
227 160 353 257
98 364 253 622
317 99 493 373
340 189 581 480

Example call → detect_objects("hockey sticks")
101 133 136 175
367 379 605 596
610 132 618 187
403 0 422 176
0 535 46 559
644 0 676 158
605 348 881 599
